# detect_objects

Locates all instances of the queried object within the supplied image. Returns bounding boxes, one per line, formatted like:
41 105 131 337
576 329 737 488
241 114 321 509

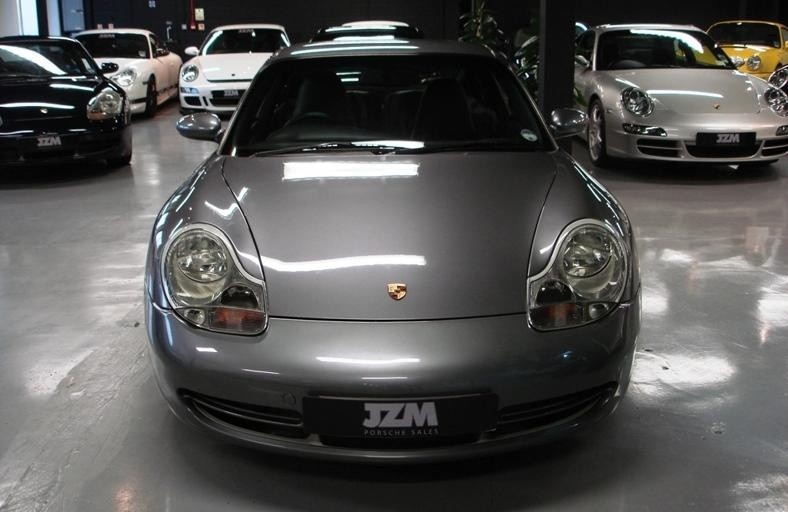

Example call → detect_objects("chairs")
280 64 481 143
605 36 669 68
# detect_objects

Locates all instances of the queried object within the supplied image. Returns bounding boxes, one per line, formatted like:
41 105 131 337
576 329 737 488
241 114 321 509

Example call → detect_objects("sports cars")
141 38 642 483
